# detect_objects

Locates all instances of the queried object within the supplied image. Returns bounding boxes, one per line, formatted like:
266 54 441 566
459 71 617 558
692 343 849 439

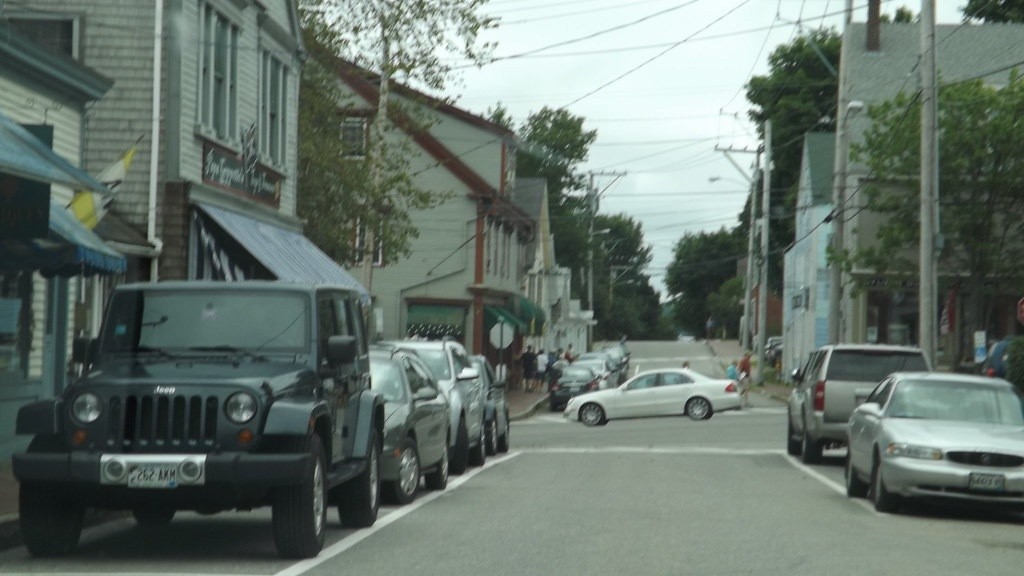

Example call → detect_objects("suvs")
10 277 388 563
786 342 934 467
395 336 488 477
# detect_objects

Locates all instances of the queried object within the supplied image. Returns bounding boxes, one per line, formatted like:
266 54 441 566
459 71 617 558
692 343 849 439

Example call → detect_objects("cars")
550 365 603 413
563 366 746 427
677 331 699 344
567 342 633 392
845 370 1024 515
364 342 452 508
471 353 511 458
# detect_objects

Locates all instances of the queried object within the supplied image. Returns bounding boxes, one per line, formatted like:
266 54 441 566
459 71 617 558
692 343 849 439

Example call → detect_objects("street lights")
709 174 759 357
586 227 612 352
825 95 864 352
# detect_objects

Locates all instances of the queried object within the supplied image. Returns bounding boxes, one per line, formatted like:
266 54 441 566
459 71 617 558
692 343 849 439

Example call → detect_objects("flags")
64 145 139 234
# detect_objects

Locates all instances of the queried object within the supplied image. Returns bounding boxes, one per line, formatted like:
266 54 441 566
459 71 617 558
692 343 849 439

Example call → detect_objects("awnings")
1 114 111 194
193 202 372 305
501 291 547 337
28 202 127 280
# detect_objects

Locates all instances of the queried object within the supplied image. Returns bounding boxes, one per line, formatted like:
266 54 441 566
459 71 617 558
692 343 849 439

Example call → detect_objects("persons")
726 352 754 410
520 343 575 393
680 362 690 386
